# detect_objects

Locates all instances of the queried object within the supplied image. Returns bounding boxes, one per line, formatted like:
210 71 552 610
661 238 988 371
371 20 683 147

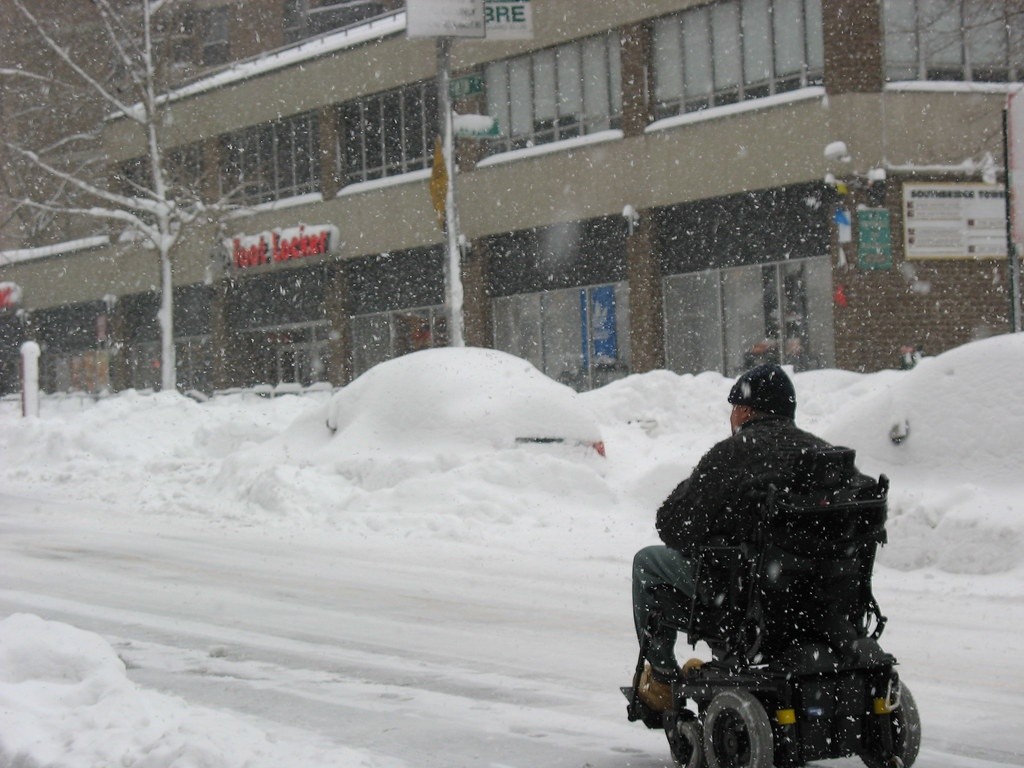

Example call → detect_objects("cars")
286 345 604 480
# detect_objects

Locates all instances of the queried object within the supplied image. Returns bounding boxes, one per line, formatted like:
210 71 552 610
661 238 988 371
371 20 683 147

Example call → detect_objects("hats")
728 363 795 417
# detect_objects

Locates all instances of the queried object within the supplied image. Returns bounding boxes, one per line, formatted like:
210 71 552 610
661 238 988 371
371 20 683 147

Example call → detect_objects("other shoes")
632 664 685 714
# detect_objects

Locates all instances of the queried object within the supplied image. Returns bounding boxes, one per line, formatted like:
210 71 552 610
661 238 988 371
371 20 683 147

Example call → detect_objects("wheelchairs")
619 445 921 768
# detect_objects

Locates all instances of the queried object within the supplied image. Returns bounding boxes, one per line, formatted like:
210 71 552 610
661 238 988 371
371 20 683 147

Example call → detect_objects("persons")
631 364 861 710
